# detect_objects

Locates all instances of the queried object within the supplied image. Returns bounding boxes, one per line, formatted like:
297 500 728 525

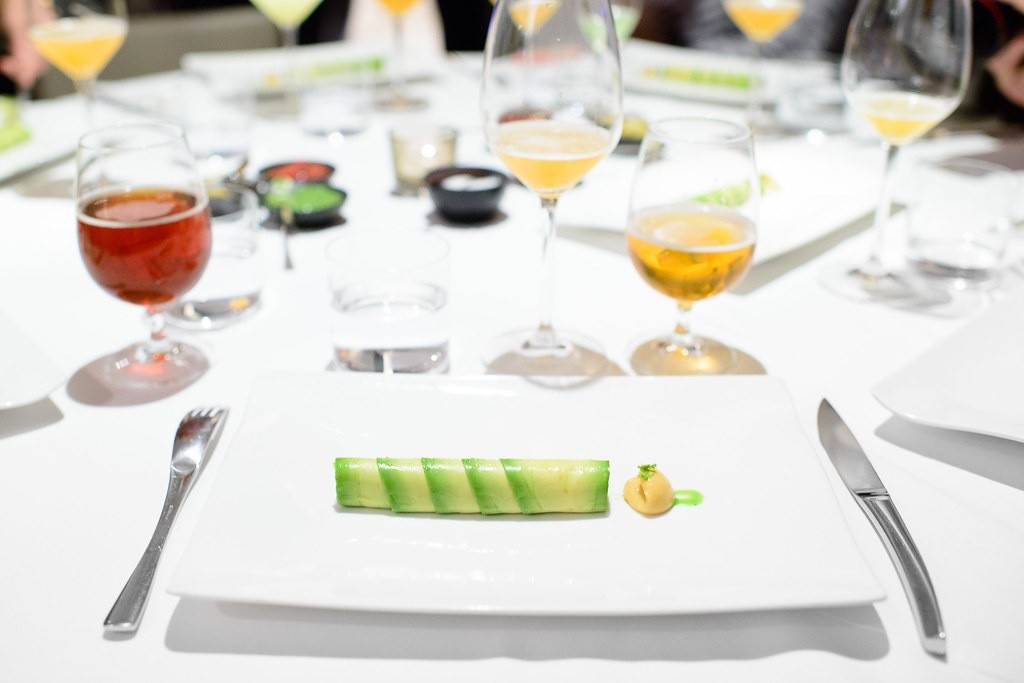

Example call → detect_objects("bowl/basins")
428 166 505 224
260 163 334 185
269 187 347 229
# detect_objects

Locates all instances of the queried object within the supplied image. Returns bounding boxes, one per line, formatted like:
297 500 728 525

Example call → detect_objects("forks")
102 403 225 630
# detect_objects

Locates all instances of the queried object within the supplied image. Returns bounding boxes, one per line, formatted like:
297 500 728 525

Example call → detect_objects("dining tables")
0 38 1024 683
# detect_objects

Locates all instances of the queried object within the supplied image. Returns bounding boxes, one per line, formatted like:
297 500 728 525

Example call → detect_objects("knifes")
817 396 947 657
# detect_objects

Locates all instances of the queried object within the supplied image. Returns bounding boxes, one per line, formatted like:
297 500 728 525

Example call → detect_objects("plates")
181 48 388 98
552 147 896 270
163 378 889 619
601 38 834 106
871 284 1024 445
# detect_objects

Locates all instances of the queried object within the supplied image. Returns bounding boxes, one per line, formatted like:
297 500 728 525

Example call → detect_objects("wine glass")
720 0 802 94
573 0 644 102
374 0 419 76
26 0 130 121
837 0 972 298
626 118 761 374
73 122 211 391
490 0 562 76
252 0 324 50
484 0 624 376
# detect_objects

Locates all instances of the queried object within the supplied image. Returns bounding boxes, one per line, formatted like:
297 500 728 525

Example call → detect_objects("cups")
165 185 267 328
389 125 459 201
324 228 452 379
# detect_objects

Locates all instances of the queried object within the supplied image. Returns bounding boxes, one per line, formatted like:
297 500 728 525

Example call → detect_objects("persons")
0 1 1024 124
